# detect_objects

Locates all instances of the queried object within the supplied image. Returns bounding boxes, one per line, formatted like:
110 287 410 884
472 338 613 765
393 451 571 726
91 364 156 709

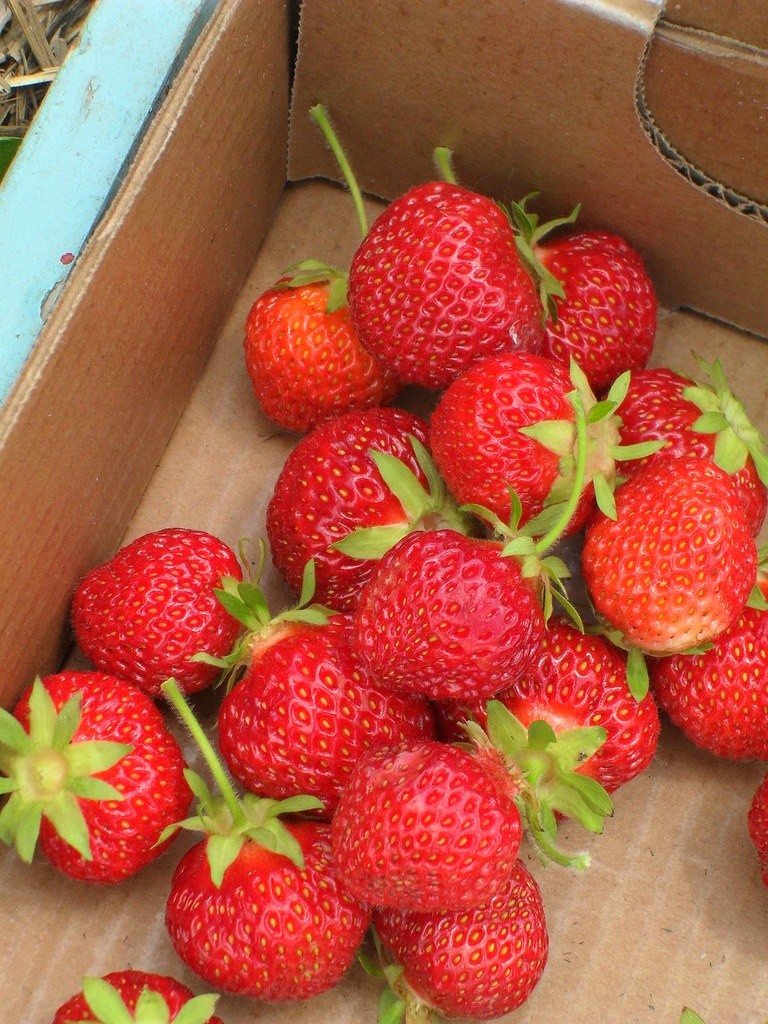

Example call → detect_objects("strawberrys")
0 177 768 1024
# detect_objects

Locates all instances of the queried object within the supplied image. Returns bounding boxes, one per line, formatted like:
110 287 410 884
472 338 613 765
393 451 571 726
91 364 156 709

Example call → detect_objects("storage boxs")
0 1 768 1022
